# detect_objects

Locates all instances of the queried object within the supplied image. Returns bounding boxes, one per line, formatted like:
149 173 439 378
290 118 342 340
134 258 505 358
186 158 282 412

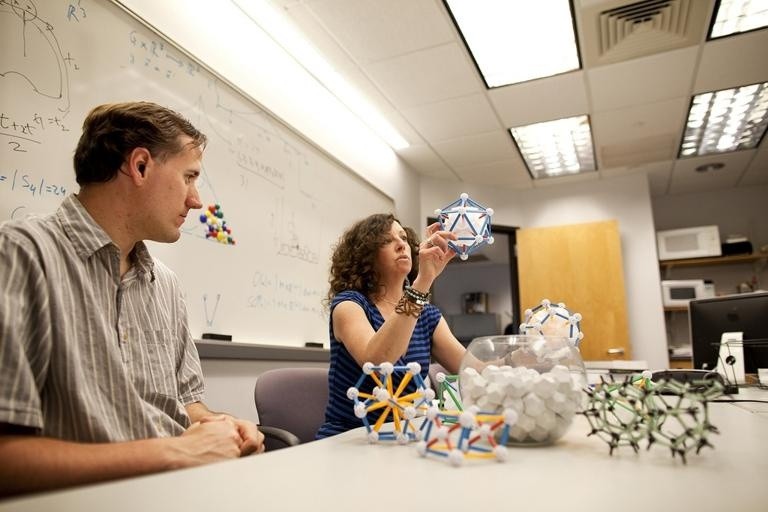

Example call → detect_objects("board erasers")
306 343 323 348
202 333 232 341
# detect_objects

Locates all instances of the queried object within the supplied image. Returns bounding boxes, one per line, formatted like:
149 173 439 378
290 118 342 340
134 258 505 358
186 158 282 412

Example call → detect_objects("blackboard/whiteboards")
0 1 397 363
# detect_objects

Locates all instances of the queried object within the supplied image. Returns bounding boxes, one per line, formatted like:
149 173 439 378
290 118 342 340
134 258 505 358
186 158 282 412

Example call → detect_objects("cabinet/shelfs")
661 250 768 371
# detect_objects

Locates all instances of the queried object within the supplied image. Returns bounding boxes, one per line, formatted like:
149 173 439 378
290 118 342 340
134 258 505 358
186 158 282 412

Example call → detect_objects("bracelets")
394 284 432 319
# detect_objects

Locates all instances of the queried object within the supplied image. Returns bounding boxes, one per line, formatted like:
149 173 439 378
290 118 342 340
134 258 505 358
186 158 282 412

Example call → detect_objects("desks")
0 383 768 511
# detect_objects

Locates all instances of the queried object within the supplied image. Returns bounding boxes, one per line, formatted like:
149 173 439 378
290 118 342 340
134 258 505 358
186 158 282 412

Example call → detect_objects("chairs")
251 367 332 452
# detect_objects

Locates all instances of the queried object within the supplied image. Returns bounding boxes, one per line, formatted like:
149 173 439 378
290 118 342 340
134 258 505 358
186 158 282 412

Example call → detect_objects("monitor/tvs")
687 292 768 388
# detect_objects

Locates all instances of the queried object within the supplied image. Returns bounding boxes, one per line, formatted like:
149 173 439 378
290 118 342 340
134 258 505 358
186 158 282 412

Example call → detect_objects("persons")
314 211 553 441
0 100 268 499
